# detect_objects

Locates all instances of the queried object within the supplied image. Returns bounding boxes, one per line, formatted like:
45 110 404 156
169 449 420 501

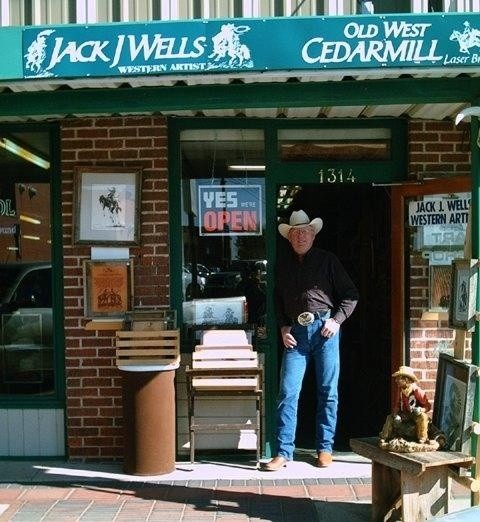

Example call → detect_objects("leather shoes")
262 456 293 471
318 451 333 467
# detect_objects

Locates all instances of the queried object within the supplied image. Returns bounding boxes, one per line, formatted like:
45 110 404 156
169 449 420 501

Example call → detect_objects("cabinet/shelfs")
188 329 266 476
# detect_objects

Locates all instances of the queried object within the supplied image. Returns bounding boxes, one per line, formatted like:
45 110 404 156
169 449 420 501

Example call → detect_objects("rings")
284 344 287 346
286 346 288 347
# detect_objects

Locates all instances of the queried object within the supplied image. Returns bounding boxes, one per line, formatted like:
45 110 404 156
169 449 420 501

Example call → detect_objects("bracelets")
333 317 341 324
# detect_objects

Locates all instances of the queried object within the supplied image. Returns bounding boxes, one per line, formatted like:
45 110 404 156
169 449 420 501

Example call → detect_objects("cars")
182 261 267 323
0 262 54 393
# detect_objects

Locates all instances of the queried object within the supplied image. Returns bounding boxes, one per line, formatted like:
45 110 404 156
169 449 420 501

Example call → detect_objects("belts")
291 311 333 327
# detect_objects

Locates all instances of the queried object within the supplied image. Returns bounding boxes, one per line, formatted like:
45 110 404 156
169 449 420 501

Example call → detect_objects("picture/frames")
426 257 477 480
71 165 178 359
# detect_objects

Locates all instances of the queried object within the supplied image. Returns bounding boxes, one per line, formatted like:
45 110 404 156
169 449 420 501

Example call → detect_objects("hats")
277 209 324 240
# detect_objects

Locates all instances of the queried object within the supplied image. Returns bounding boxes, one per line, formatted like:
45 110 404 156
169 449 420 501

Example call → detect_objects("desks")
116 362 179 476
349 434 475 522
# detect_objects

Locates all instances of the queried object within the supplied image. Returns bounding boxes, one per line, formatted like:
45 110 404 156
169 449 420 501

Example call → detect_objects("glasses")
291 227 315 235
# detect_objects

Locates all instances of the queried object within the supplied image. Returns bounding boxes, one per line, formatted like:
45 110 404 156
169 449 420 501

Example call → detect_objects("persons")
258 211 361 470
379 365 432 443
